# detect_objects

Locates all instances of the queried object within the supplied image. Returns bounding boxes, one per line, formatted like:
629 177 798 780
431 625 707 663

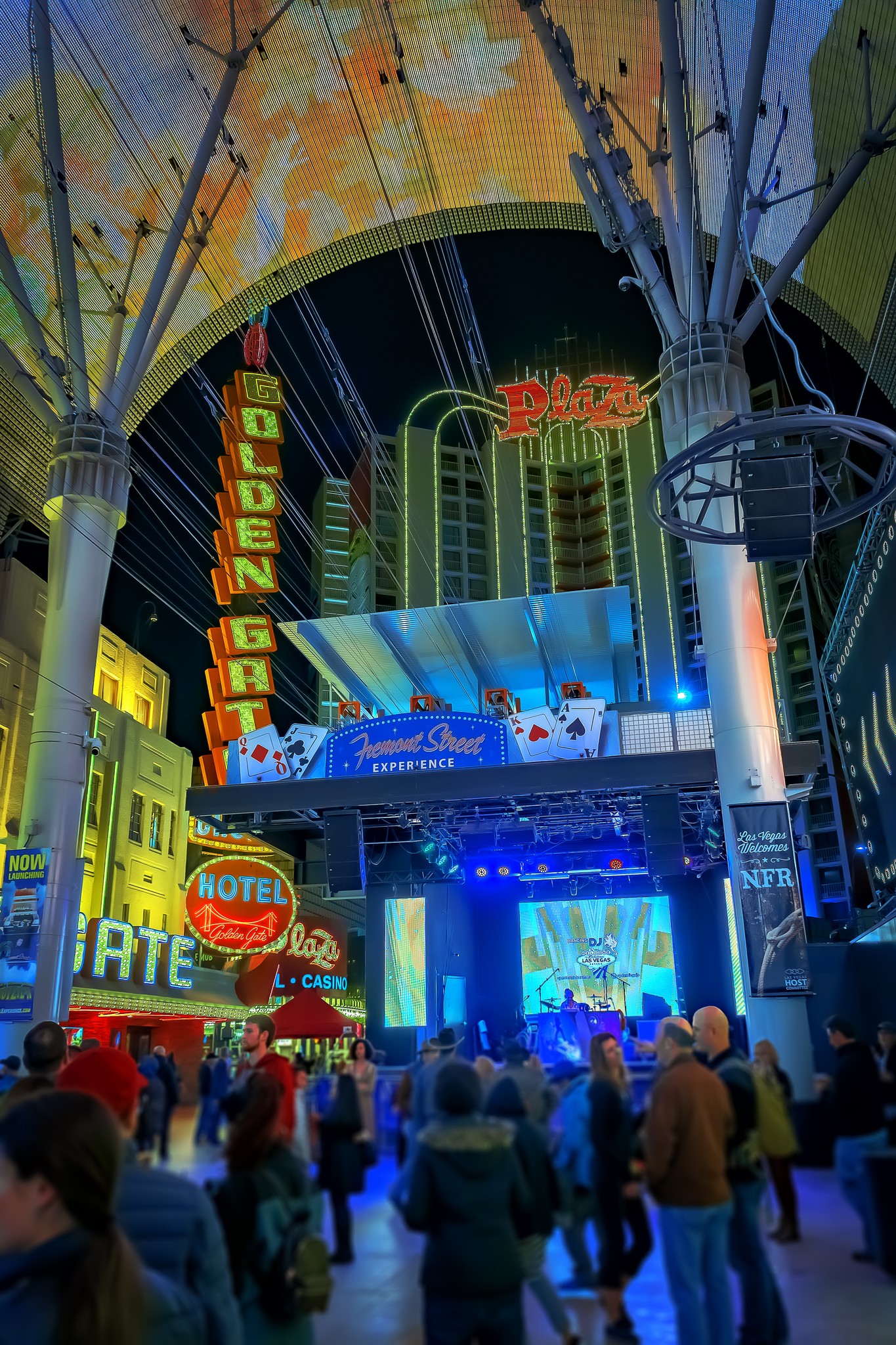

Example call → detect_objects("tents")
269 987 362 1039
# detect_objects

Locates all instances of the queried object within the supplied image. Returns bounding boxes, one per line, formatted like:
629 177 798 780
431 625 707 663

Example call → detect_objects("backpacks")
258 1162 331 1316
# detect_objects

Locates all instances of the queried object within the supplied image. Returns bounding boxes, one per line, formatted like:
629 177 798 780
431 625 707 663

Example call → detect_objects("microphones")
558 968 560 972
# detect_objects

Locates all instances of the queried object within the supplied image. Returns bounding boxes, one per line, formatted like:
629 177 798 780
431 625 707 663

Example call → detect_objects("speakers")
635 1019 661 1043
641 789 686 876
323 809 365 896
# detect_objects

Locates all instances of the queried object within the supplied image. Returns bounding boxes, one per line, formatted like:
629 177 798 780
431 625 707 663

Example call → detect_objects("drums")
575 1002 590 1012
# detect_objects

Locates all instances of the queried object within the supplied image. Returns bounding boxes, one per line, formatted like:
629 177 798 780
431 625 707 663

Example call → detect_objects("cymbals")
587 995 602 998
593 1002 606 1004
540 1000 559 1010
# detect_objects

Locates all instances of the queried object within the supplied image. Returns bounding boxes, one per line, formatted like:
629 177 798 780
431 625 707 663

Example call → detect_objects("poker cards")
547 696 607 760
237 723 291 784
280 723 329 780
507 705 562 763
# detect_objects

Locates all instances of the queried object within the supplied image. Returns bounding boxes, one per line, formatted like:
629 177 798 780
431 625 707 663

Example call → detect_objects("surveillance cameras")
91 737 102 756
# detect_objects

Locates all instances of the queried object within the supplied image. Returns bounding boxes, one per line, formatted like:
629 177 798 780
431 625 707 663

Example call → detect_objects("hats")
59 1048 149 1113
429 1030 465 1051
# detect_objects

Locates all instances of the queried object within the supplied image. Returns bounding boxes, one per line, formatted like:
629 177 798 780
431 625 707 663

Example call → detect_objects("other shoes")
853 1249 877 1262
606 1319 638 1345
771 1222 797 1244
561 1278 598 1295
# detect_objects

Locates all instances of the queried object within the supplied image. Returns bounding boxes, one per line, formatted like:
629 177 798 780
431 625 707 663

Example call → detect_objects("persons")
343 1038 377 1141
875 1020 896 1147
814 1014 888 1264
136 1045 180 1162
284 1065 311 1161
56 1047 243 1345
394 1027 594 1345
0 1020 69 1118
0 1088 202 1345
750 1039 800 1243
561 988 578 1009
219 1015 296 1143
693 1006 790 1345
584 1032 654 1345
204 1085 316 1345
0 1055 21 1093
317 1073 366 1265
642 1016 735 1345
